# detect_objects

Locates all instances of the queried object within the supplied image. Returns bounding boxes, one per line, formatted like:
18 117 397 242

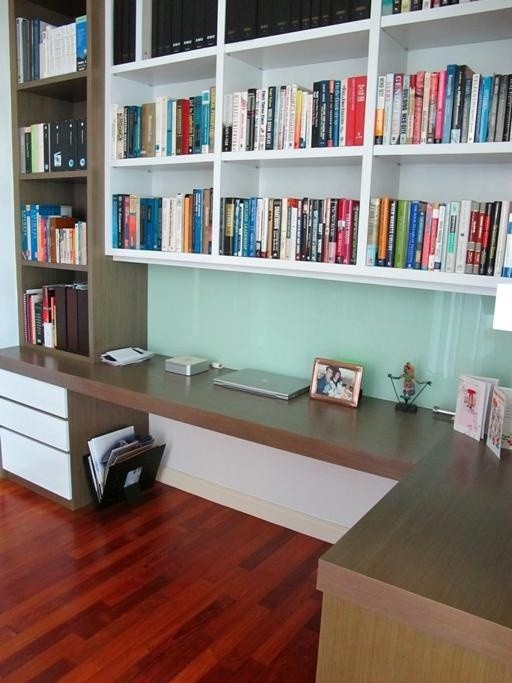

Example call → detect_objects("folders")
77 289 89 357
114 0 370 62
83 444 166 510
54 286 68 351
66 287 79 353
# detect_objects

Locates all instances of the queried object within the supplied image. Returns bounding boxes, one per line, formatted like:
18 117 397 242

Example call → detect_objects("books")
113 1 466 65
14 14 88 362
112 188 510 278
113 64 512 159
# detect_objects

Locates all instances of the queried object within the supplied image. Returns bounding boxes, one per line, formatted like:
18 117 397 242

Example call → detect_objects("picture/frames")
309 357 363 409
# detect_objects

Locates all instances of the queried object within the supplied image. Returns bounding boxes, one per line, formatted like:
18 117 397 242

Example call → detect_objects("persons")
323 370 343 394
316 365 334 393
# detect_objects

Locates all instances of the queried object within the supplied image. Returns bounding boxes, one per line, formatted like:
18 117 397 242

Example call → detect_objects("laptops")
212 369 311 401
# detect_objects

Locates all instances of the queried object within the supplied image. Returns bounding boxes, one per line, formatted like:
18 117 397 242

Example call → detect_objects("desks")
1 333 512 683
